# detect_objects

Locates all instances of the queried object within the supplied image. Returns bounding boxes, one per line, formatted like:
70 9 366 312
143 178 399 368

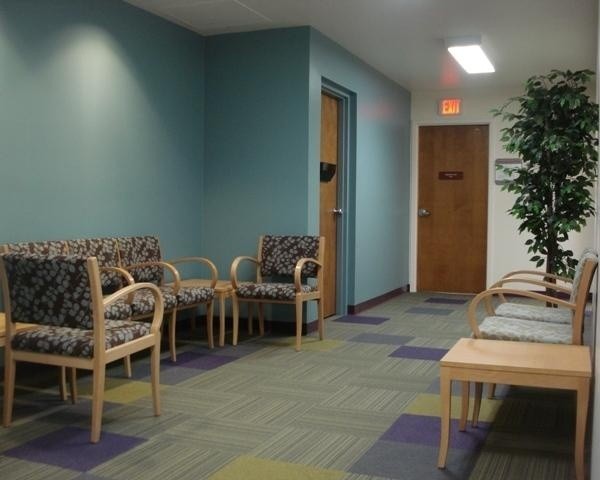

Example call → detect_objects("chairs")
485 246 598 324
1 253 165 444
66 237 180 378
230 234 325 352
468 252 598 429
1 240 68 400
116 235 218 362
